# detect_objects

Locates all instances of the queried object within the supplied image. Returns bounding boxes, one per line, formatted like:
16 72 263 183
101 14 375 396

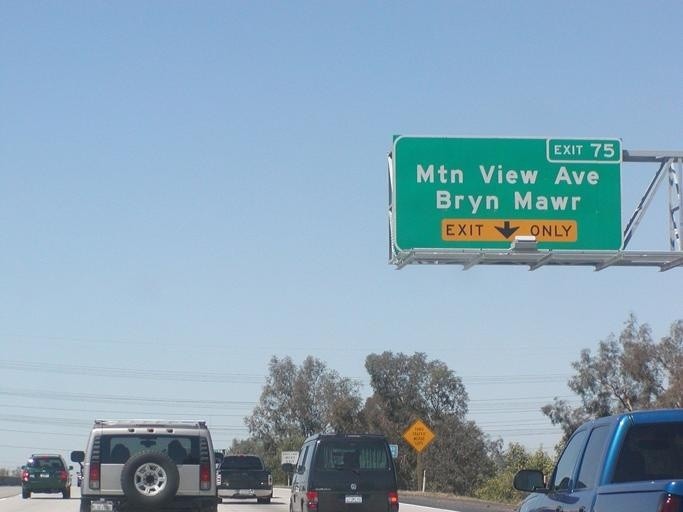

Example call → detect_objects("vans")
282 432 399 511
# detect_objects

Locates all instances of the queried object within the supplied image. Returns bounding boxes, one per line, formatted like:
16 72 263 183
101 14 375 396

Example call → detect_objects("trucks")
213 449 225 469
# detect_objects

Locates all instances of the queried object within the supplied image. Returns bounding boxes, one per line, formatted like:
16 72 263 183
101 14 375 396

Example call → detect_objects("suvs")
19 452 74 500
76 465 84 488
72 418 219 512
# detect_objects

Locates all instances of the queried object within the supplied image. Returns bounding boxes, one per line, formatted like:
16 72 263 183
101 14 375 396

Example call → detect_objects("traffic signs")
393 133 626 252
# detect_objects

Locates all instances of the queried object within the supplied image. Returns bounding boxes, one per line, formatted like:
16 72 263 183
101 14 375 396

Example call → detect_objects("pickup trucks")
514 409 683 512
216 454 275 505
